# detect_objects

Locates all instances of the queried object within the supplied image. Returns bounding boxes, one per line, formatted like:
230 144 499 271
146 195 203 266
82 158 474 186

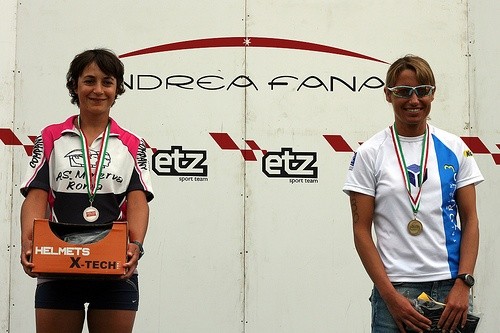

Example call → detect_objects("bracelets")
130 241 145 260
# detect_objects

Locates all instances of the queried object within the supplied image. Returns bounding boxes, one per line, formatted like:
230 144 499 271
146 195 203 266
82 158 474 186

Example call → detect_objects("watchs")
456 273 475 288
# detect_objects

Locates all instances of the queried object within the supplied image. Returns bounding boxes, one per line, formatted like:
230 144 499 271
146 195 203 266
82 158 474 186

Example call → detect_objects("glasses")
388 85 434 98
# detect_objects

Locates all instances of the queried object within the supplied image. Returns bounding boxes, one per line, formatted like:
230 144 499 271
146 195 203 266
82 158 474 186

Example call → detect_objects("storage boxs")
29 218 128 280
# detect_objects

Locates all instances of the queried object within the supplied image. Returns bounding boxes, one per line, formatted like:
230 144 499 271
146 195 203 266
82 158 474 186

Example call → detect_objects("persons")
342 54 485 333
19 49 154 333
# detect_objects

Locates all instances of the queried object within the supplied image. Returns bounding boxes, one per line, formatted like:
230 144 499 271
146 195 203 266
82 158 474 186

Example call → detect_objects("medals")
407 218 423 236
83 205 99 222
